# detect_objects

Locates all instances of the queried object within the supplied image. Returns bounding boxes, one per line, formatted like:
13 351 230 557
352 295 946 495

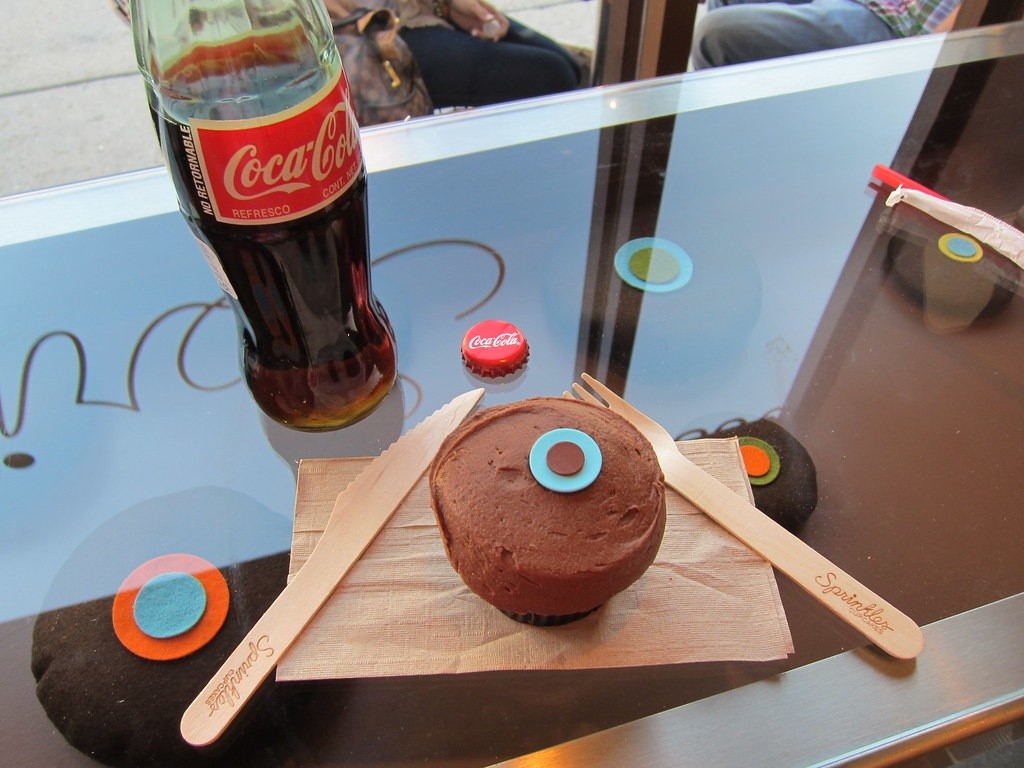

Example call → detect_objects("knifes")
181 388 488 748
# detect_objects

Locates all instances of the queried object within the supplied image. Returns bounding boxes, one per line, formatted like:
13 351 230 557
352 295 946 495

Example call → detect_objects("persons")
690 0 963 71
324 0 583 105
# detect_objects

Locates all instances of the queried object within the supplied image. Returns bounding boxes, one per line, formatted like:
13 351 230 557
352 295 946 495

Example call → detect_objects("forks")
557 373 924 658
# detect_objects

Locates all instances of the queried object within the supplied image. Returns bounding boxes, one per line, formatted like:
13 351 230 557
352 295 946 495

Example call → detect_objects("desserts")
428 396 666 627
29 487 353 768
709 417 818 535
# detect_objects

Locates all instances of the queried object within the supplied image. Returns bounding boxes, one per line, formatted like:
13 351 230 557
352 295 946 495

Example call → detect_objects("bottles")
131 0 396 433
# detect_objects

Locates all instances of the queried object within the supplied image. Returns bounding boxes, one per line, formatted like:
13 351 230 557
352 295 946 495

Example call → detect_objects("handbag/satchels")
330 7 434 127
